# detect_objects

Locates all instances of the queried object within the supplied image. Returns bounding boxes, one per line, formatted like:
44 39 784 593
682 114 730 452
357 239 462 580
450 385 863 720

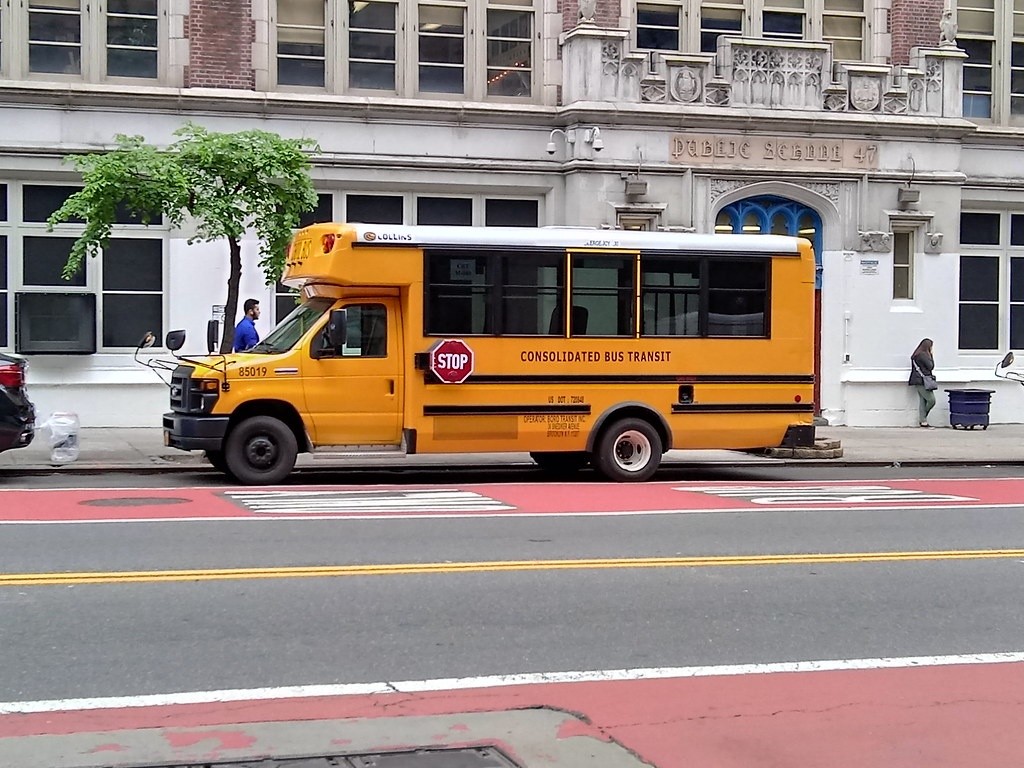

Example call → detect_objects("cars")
0 351 38 454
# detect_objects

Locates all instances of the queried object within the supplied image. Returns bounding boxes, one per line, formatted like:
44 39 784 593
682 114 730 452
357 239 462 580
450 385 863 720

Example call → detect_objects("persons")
234 298 260 352
908 339 936 428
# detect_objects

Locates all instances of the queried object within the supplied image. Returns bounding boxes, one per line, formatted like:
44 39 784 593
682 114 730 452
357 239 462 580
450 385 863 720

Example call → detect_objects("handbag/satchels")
923 375 938 390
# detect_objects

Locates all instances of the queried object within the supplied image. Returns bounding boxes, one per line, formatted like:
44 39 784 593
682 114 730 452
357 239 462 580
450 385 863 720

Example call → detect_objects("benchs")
548 304 590 333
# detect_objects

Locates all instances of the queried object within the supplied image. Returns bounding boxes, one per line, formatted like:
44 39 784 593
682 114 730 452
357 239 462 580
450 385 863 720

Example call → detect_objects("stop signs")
433 340 475 384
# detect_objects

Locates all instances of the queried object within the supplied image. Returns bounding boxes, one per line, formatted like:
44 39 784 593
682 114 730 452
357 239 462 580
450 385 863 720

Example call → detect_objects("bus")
134 221 816 487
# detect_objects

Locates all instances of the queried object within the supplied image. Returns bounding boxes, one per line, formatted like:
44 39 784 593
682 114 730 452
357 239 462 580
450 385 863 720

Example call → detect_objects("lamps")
624 148 649 196
584 125 603 152
897 156 919 204
547 128 575 154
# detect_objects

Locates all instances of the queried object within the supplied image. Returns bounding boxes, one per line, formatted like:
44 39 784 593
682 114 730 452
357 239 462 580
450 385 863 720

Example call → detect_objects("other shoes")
920 424 935 430
